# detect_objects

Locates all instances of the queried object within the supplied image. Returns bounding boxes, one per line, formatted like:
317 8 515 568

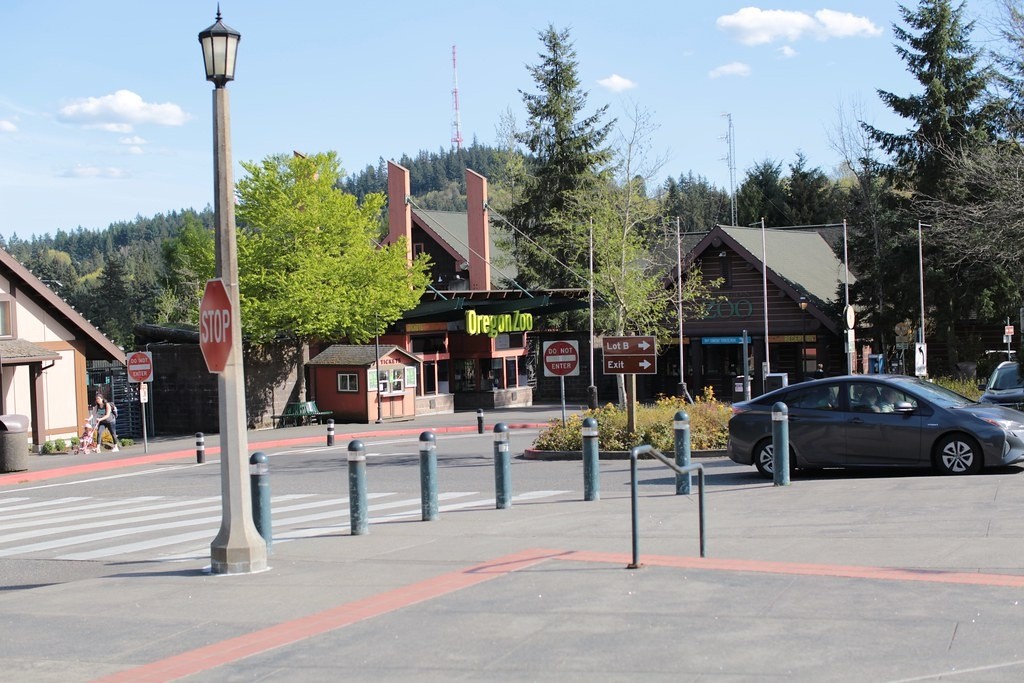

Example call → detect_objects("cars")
727 375 1024 475
978 361 1024 410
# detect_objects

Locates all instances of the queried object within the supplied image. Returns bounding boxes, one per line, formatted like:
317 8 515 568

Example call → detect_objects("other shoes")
93 448 100 453
112 448 119 452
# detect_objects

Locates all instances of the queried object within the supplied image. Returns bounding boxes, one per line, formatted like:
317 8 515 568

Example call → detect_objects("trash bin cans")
734 375 753 404
0 415 29 473
766 373 788 392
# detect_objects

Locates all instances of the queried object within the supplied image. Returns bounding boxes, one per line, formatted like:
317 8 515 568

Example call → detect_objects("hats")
83 424 91 428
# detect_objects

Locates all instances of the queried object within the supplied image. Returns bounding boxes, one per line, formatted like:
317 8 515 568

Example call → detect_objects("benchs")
270 400 334 427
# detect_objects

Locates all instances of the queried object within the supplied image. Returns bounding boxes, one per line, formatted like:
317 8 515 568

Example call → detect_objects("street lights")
198 1 266 574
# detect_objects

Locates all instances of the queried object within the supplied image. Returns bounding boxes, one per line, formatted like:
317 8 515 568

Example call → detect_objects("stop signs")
127 352 153 382
198 281 233 374
543 341 579 377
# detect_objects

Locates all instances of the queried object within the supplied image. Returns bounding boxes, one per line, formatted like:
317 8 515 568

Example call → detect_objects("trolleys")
73 418 100 455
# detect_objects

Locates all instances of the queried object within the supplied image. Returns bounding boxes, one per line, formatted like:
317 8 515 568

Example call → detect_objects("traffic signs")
602 336 656 375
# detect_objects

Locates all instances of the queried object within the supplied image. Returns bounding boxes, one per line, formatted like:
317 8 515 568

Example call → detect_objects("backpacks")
105 402 118 418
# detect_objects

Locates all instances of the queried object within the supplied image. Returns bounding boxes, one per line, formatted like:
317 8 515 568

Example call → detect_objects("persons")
817 388 833 407
877 387 899 411
81 424 92 448
85 393 119 453
814 364 824 379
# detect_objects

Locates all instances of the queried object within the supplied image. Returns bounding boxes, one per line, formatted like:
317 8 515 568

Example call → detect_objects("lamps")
719 250 726 257
